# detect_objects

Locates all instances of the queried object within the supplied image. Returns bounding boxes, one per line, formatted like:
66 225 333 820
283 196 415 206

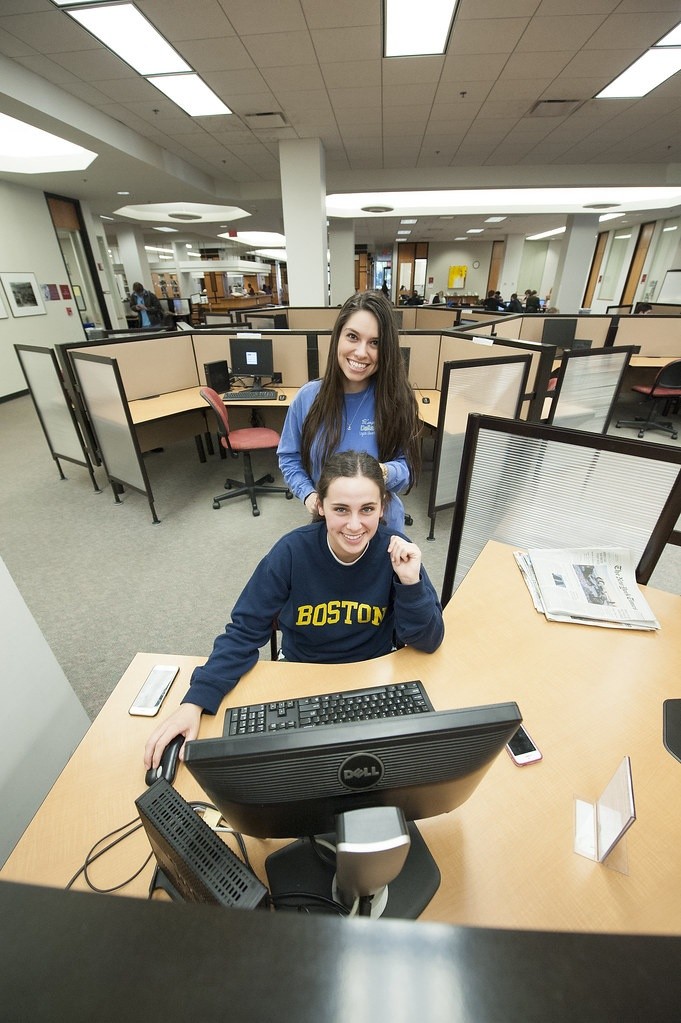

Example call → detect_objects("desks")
0 539 681 936
127 314 190 324
128 386 513 435
551 356 681 372
433 296 478 304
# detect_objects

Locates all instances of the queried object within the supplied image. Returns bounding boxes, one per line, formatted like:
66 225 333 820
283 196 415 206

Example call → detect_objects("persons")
129 282 162 328
381 280 446 306
276 288 424 535
483 289 541 314
143 449 444 771
633 302 652 315
247 283 273 298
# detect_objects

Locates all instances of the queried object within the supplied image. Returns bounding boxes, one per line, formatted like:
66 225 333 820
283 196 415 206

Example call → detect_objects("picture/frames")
0 272 47 318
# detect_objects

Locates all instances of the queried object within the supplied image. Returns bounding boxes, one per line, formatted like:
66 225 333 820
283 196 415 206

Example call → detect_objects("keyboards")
222 680 436 738
222 392 278 401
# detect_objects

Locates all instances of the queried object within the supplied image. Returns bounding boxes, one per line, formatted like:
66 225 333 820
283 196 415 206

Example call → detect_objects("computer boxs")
135 776 270 912
203 360 230 395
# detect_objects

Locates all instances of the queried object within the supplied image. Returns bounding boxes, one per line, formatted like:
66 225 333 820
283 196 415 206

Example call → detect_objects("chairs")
199 387 293 516
616 359 681 439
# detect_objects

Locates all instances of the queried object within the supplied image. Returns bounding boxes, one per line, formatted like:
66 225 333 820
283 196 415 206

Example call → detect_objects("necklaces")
341 385 370 430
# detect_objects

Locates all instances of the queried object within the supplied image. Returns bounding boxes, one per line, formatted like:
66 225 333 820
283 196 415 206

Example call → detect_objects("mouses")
279 395 286 401
145 734 185 786
422 397 430 403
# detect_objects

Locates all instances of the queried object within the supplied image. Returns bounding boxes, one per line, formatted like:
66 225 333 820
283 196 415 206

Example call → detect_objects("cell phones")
505 724 542 766
129 665 181 717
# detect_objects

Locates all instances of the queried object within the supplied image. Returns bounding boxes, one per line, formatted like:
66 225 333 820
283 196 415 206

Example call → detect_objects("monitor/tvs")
185 702 522 922
460 319 480 327
230 338 275 393
159 293 208 315
400 346 410 380
542 318 578 355
